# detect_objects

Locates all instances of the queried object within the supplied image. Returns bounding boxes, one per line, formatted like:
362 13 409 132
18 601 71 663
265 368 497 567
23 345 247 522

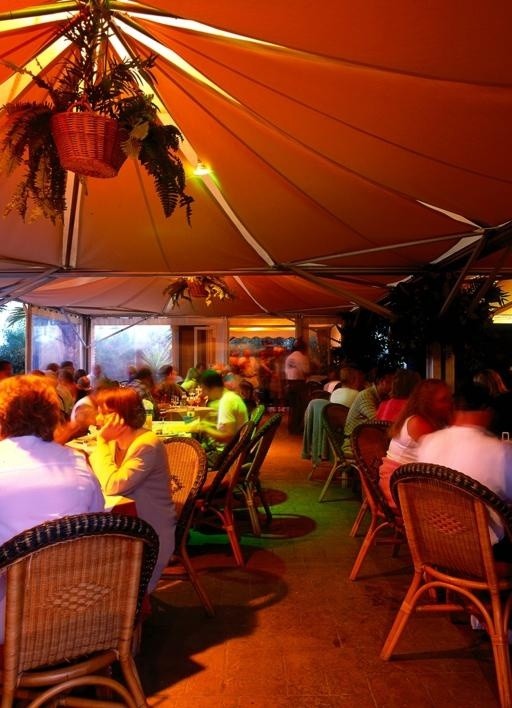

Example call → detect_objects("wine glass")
159 409 169 425
172 386 203 411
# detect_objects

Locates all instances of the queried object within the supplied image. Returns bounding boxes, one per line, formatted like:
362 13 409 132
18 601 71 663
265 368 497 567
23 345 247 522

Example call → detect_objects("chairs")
382 465 512 708
0 510 162 706
3 384 512 598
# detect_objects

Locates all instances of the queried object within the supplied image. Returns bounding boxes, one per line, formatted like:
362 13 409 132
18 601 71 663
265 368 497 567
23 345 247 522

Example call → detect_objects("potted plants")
0 0 194 228
162 275 235 310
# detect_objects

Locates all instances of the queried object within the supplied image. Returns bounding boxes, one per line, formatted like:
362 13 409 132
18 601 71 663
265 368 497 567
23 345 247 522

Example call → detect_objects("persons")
257 339 312 435
320 354 511 642
0 348 258 590
311 333 328 375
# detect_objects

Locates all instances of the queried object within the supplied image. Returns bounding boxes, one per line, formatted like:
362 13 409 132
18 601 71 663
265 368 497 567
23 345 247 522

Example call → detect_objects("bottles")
140 397 153 428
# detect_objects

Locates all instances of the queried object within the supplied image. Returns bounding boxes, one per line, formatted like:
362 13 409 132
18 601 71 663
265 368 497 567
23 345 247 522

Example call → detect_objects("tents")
0 0 512 325
